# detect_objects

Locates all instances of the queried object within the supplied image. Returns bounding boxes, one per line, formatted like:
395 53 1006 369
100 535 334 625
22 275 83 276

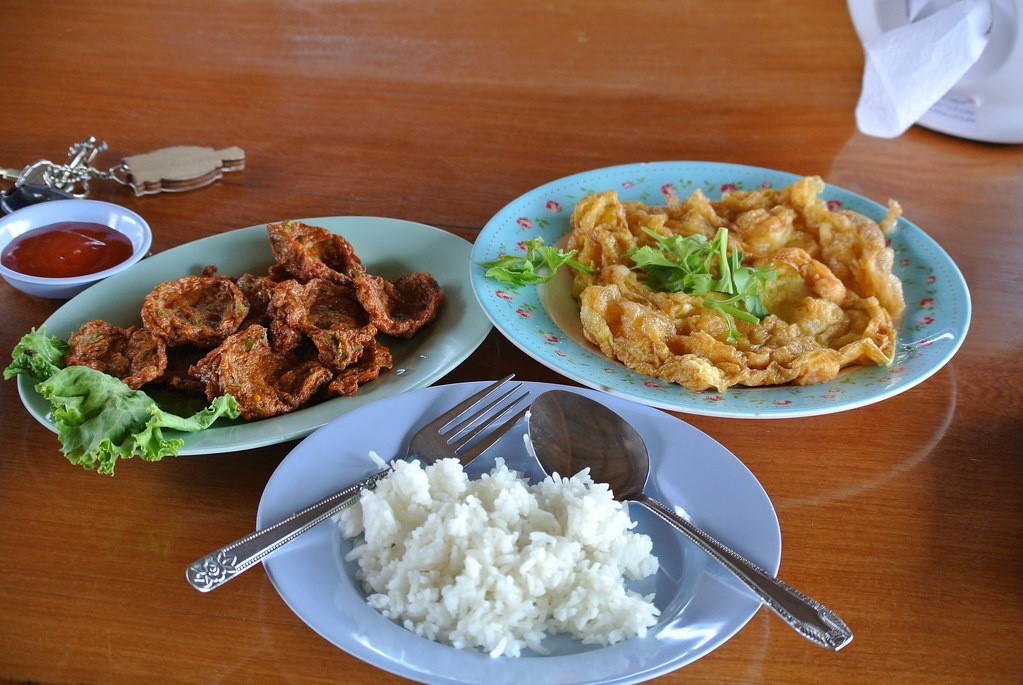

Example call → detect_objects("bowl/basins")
0 198 153 299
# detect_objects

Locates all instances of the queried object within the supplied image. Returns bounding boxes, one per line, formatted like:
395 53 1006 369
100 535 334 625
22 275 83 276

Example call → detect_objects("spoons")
528 389 853 652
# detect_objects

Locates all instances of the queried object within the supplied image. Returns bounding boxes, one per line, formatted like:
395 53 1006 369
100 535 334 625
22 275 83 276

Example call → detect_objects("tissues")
847 0 1023 145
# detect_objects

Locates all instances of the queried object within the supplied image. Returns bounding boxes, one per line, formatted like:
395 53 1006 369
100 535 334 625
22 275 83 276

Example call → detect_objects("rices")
338 449 661 656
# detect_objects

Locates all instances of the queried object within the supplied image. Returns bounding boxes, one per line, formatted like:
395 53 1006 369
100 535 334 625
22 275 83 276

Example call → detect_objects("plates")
470 160 972 419
256 380 784 685
17 216 493 457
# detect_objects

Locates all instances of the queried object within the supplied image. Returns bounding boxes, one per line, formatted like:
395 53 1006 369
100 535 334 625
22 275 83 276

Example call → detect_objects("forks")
183 373 533 593
